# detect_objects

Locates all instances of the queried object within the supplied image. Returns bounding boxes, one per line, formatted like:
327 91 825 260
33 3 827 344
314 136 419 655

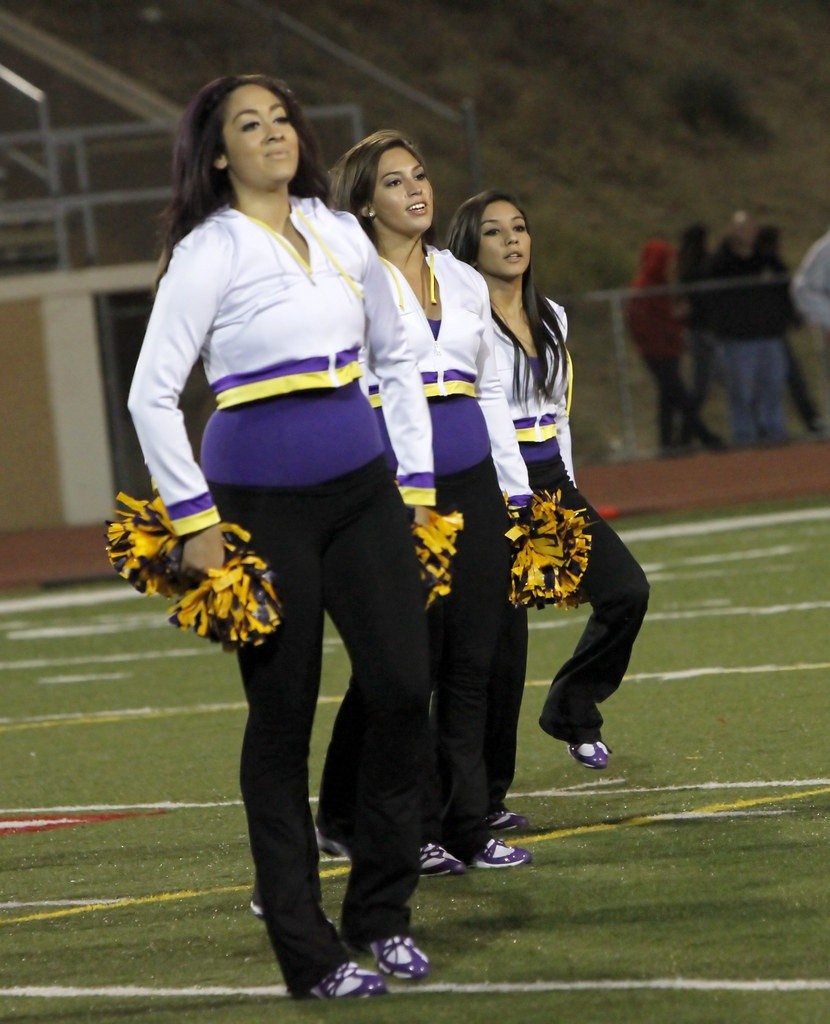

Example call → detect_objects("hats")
750 221 781 245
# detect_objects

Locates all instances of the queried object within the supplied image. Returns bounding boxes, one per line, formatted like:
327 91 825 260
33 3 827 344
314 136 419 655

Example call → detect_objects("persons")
447 189 650 833
125 72 437 1005
625 209 830 460
314 130 531 880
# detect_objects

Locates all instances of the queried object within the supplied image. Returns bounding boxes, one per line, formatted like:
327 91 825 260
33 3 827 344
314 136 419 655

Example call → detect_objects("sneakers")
364 934 430 978
309 959 386 1001
567 739 609 768
418 843 466 877
479 809 529 830
312 826 349 859
249 897 335 926
467 837 532 868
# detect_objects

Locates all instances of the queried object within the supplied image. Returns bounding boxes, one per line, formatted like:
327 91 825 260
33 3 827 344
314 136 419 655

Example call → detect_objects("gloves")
509 505 533 527
